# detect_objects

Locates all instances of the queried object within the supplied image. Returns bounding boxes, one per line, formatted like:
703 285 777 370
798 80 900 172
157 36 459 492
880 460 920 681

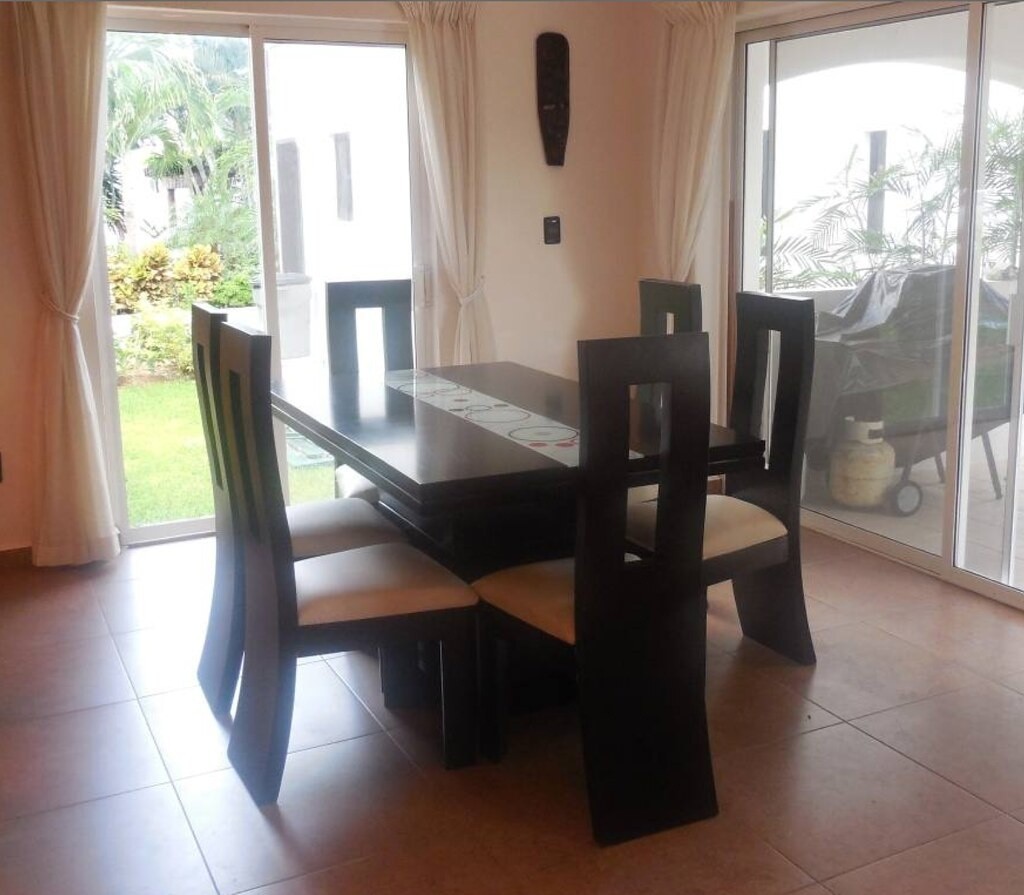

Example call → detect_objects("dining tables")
270 358 770 738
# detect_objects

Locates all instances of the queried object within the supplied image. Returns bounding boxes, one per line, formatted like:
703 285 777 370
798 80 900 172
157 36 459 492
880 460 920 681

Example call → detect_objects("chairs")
465 330 723 851
214 321 485 804
628 276 725 503
324 278 414 503
628 289 818 665
189 299 442 724
